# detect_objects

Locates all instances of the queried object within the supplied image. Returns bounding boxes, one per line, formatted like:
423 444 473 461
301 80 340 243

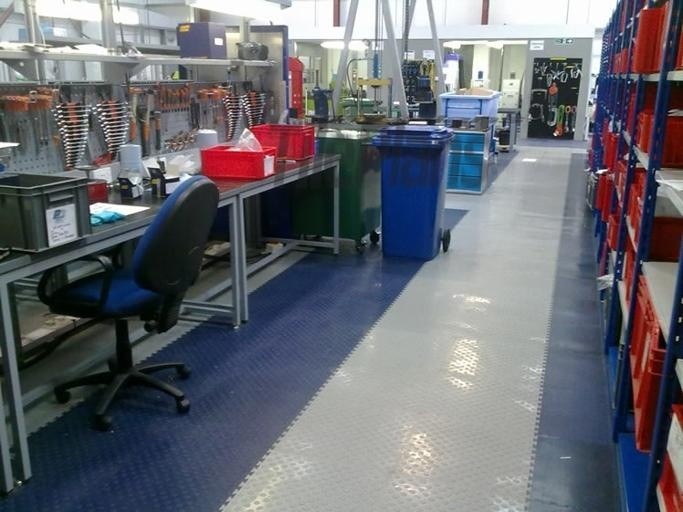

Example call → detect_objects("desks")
133 153 342 326
1 180 242 495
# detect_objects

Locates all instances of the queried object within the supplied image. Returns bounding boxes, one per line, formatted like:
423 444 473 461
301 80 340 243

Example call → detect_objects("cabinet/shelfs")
585 0 682 512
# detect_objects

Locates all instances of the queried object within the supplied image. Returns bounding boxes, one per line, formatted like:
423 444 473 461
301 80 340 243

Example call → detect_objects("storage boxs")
249 121 318 159
175 22 227 59
201 143 279 181
1 170 98 257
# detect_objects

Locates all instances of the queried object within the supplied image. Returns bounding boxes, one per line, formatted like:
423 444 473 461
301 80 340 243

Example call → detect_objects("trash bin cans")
372 124 457 260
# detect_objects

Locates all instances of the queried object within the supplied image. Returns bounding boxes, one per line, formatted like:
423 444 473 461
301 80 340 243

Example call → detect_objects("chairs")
31 174 220 434
